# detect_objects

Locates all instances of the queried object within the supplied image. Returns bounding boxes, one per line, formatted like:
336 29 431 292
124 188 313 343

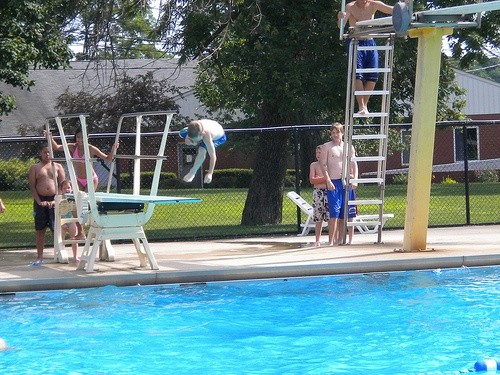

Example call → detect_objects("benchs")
287 191 395 238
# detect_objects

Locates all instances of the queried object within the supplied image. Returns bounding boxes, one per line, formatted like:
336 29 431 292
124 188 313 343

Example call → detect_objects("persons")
310 145 336 246
337 1 409 114
42 128 119 240
28 143 65 267
319 122 358 246
183 118 227 184
42 180 79 261
0 197 5 214
